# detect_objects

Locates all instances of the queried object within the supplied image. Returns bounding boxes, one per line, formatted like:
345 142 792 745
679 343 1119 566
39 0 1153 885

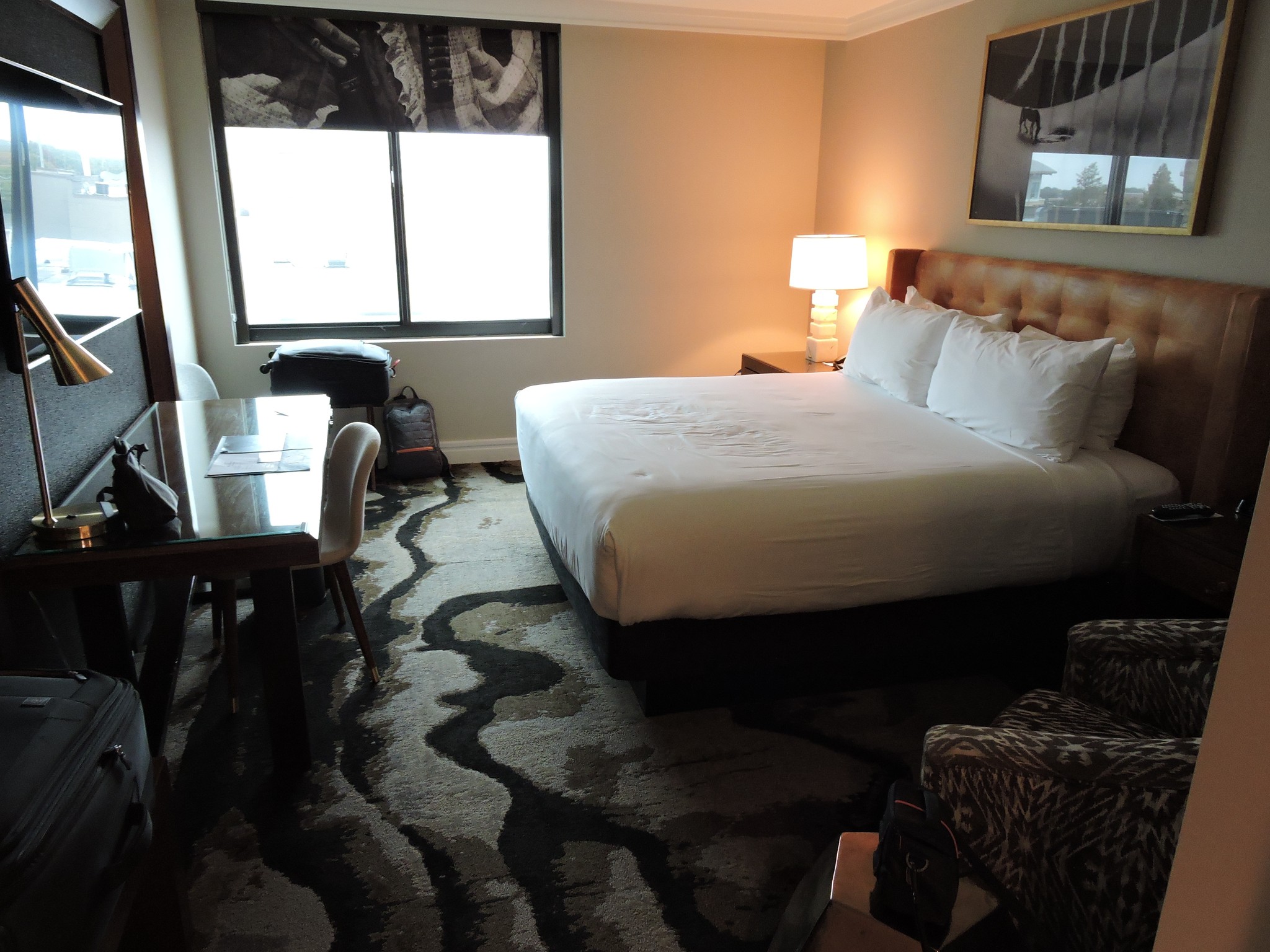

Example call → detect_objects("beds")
513 248 1269 714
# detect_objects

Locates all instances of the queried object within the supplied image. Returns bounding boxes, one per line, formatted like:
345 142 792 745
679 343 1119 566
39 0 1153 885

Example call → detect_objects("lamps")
787 234 869 363
8 276 122 540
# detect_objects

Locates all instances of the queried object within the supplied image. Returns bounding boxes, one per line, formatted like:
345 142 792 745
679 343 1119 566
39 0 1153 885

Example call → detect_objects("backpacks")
382 386 450 481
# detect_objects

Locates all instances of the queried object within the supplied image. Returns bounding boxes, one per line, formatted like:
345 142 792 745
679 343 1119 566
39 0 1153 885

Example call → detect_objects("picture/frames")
966 0 1243 235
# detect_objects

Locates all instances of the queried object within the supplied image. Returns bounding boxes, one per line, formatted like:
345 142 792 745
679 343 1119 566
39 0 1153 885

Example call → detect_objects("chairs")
177 363 218 402
227 421 381 718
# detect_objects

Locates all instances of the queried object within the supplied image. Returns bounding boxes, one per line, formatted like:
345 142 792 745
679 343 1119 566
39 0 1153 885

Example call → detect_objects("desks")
330 402 386 490
15 394 332 781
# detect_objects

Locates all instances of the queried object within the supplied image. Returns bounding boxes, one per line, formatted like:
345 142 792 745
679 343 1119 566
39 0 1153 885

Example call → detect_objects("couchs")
917 620 1233 952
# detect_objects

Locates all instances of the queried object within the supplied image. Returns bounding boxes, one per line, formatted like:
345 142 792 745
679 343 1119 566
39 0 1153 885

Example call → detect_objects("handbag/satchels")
94 435 183 539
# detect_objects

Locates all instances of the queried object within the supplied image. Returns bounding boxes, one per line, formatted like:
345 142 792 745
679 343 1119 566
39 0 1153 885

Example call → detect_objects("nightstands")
1128 511 1249 618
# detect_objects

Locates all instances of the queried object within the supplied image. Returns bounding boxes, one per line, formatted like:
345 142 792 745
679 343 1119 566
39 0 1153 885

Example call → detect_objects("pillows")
841 286 964 407
1016 323 1139 453
925 314 1119 462
904 285 1012 333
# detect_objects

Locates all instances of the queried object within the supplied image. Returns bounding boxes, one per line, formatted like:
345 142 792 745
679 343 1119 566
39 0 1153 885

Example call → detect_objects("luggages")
0 670 157 952
259 339 401 407
